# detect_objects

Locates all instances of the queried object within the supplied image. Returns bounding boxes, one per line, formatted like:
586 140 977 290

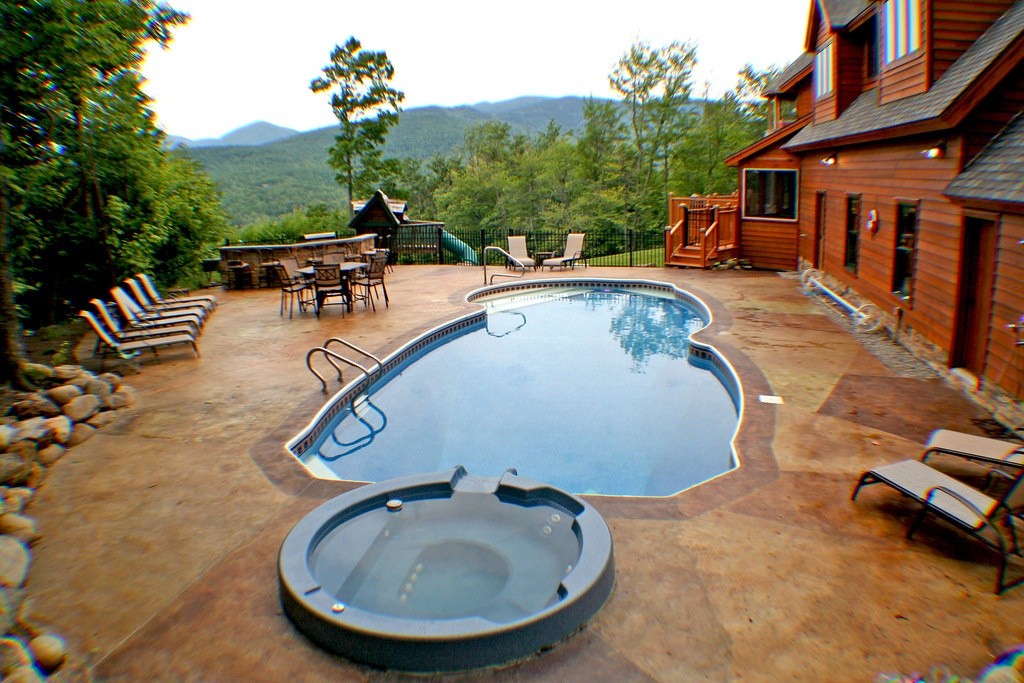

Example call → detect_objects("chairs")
225 250 253 291
89 298 195 346
78 310 201 370
123 278 213 318
114 286 206 327
920 430 1024 490
850 459 1024 594
273 235 393 320
108 288 203 334
110 343 140 375
542 233 587 271
258 248 281 290
134 273 218 311
505 236 536 272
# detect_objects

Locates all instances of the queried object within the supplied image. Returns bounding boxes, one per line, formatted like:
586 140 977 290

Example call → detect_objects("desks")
536 252 553 269
294 262 368 315
